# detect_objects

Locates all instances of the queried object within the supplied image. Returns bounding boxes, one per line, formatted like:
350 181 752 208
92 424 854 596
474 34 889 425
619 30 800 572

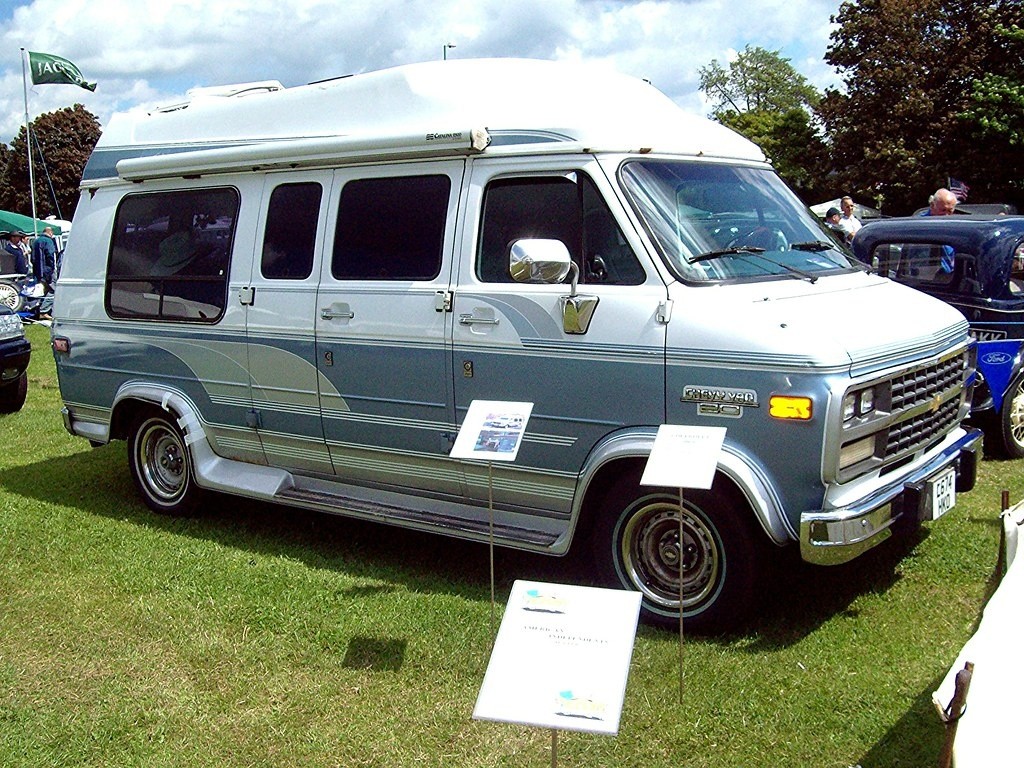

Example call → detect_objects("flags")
949 177 970 202
26 50 97 93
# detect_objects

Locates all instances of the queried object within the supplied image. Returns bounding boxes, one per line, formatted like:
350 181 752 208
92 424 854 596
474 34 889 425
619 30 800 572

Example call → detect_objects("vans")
51 55 984 637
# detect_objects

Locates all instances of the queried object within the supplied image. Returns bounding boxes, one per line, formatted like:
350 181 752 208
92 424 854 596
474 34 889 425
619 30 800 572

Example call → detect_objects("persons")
5 228 66 324
836 196 862 242
997 211 1024 272
823 207 851 249
909 188 956 283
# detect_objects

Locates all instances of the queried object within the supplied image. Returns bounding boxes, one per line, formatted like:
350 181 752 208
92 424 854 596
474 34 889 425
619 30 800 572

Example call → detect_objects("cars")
0 230 30 314
568 199 1024 462
0 305 32 415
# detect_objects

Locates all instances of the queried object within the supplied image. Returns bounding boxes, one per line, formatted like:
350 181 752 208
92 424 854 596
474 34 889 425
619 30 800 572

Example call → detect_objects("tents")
0 209 62 275
